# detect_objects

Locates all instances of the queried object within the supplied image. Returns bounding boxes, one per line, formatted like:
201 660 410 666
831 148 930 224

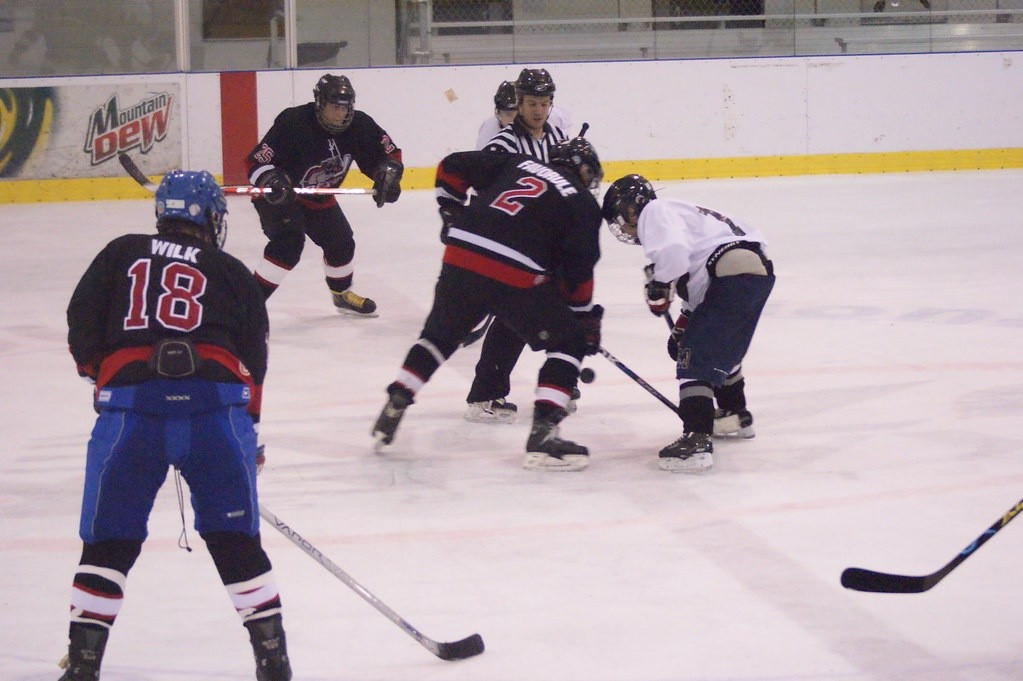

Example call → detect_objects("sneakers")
711 406 756 438
523 417 590 471
372 391 413 453
464 393 519 425
658 418 712 471
244 615 292 681
57 620 108 681
331 287 379 317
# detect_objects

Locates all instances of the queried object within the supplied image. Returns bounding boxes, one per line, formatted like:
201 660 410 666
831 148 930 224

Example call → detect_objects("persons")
244 73 404 318
59 168 293 681
474 80 574 151
464 68 581 424
375 137 605 471
602 173 779 471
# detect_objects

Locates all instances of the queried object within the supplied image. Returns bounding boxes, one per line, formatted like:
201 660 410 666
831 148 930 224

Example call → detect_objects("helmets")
515 69 555 100
154 168 228 249
311 72 355 135
602 173 655 245
494 81 520 111
550 138 604 191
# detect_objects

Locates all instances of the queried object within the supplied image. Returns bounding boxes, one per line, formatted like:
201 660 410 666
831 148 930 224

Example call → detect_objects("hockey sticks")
456 120 591 350
118 151 379 200
660 300 736 424
585 337 754 437
255 502 486 663
839 499 1023 595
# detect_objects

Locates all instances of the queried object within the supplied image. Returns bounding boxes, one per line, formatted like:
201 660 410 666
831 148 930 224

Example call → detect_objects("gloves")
437 197 464 244
260 176 293 222
370 158 404 208
646 279 673 317
570 304 604 357
666 309 692 361
